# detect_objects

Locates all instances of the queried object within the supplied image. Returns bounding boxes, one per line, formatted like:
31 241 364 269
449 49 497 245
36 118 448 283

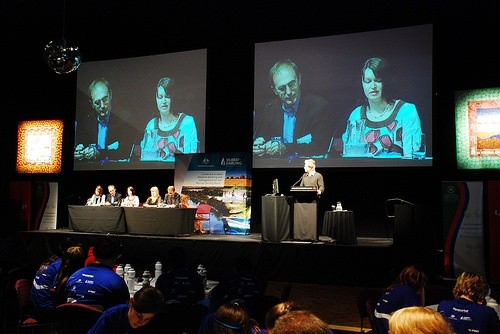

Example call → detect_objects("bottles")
337 202 341 210
197 265 207 290
142 270 151 289
155 261 163 281
127 276 134 292
116 266 123 277
128 269 135 285
124 264 131 284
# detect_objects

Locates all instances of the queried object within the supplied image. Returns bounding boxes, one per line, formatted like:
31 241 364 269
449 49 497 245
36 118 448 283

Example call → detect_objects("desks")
68 205 195 236
261 196 293 241
323 211 357 244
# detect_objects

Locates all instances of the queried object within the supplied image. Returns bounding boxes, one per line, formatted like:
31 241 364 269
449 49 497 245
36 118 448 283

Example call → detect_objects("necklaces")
161 116 176 127
369 104 389 118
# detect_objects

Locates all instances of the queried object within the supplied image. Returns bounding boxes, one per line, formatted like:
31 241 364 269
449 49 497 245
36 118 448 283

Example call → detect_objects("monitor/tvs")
272 178 279 196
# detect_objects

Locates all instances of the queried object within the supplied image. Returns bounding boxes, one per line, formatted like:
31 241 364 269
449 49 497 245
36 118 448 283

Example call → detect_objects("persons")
122 187 140 207
87 185 106 206
224 190 251 212
29 244 85 323
181 194 206 233
373 265 500 334
255 60 336 158
140 77 197 161
87 285 164 334
64 234 130 309
213 301 333 334
158 186 180 208
74 78 137 161
15 279 38 325
143 187 161 208
105 185 121 206
300 159 324 197
342 56 426 159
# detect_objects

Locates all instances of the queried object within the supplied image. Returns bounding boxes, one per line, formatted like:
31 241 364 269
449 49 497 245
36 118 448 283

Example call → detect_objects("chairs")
355 290 383 334
56 304 103 334
15 278 37 325
195 204 212 236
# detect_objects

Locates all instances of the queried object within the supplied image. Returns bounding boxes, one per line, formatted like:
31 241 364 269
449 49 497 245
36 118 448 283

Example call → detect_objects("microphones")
324 129 338 159
127 123 141 163
292 175 303 187
